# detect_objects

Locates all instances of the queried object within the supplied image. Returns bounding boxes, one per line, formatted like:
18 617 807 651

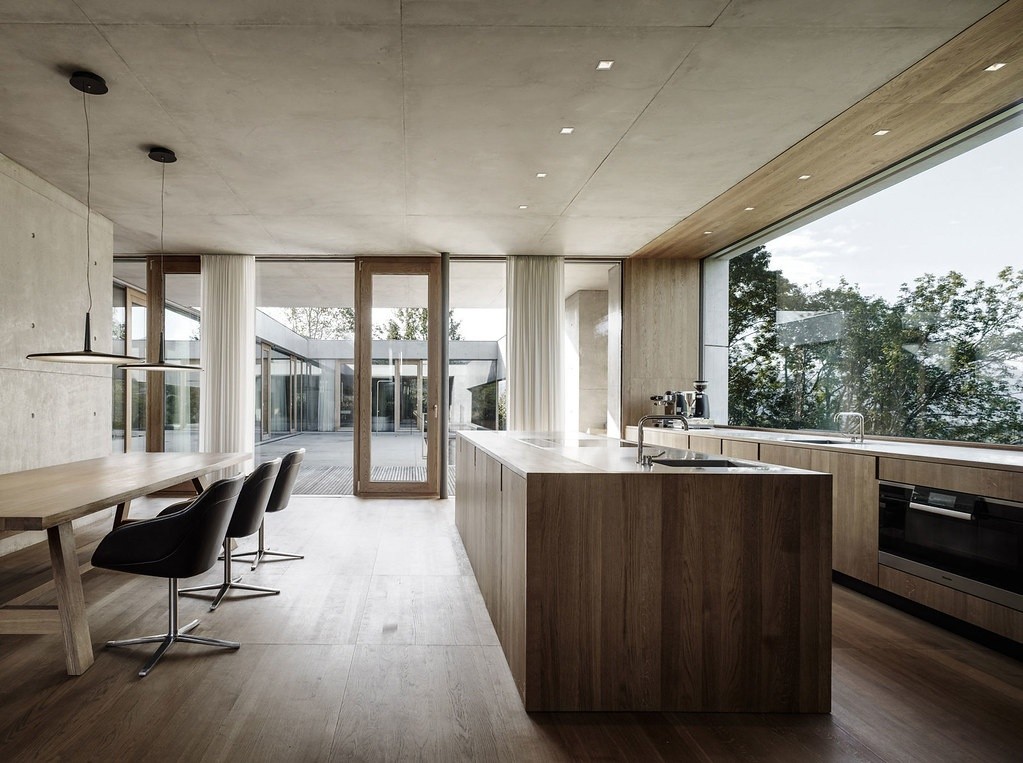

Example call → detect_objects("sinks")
651 459 763 468
782 438 867 444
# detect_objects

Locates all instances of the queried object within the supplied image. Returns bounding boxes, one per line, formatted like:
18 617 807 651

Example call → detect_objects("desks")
0 451 253 675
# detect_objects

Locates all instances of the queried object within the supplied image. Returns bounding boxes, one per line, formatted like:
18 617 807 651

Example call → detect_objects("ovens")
878 480 1023 613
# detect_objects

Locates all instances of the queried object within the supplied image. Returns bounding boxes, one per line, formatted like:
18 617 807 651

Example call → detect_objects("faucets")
834 412 864 442
637 414 689 461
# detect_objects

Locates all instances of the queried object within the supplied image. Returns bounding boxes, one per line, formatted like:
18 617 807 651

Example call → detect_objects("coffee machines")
650 380 714 429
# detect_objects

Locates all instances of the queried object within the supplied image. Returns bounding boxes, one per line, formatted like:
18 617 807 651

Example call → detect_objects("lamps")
117 145 202 372
25 72 145 364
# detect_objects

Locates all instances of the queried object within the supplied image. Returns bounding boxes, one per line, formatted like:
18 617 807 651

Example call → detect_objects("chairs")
178 456 283 609
89 471 244 677
217 447 306 570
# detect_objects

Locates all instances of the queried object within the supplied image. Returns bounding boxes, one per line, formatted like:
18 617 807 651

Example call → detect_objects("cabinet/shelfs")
456 433 832 713
625 425 878 588
